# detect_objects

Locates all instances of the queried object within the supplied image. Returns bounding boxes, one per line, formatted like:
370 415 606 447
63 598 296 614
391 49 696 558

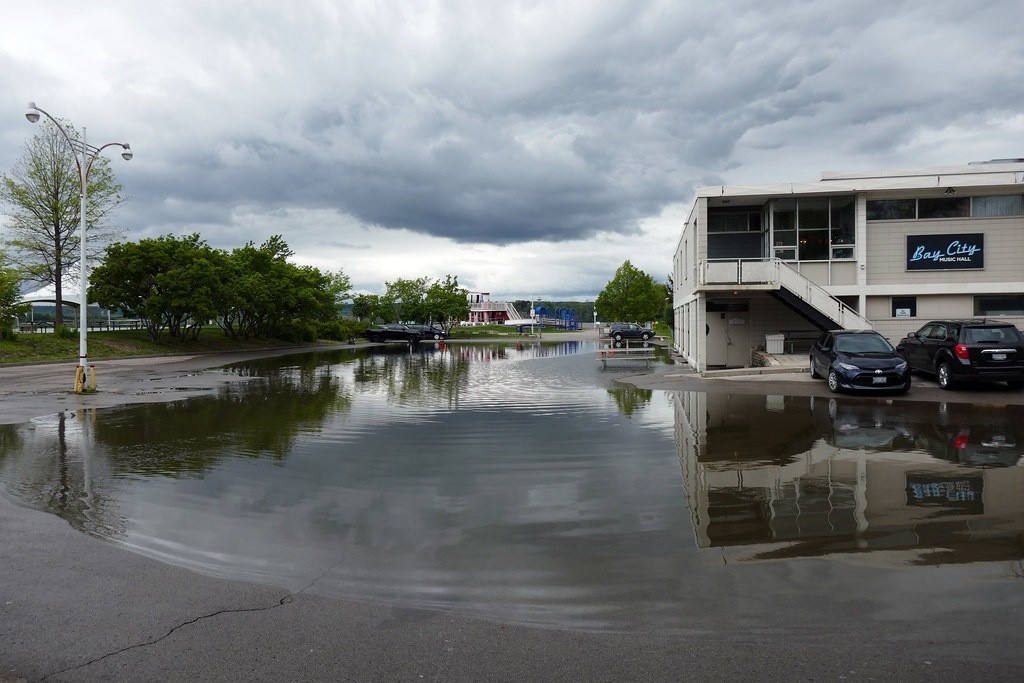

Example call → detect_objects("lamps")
945 188 956 196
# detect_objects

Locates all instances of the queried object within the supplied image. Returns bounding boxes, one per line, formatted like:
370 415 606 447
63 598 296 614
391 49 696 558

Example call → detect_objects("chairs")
991 332 1001 339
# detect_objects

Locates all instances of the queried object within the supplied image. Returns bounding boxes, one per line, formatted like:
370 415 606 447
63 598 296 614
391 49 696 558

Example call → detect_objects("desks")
785 340 801 354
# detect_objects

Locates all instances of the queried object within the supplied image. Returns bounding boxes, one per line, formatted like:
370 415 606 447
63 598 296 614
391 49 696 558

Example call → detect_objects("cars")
365 323 425 343
814 400 909 451
408 325 448 339
896 318 1024 389
918 406 1024 468
810 329 910 392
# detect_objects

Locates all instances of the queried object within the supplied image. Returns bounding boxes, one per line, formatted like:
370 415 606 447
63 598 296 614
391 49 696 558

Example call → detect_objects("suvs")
610 322 656 341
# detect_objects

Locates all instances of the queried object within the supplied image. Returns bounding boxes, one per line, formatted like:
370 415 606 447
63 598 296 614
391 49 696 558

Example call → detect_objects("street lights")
529 297 541 335
26 101 134 392
